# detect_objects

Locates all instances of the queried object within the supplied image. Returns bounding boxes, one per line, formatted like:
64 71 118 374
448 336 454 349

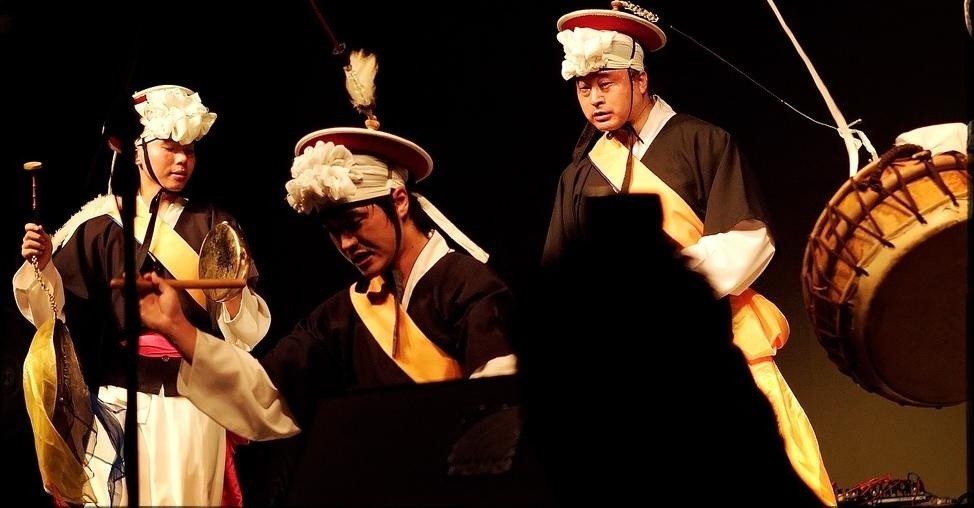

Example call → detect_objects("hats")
556 9 667 80
285 127 434 214
101 85 217 147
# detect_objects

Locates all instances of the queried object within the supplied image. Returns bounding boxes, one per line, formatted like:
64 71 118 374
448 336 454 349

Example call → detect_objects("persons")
109 128 516 442
492 204 826 506
10 84 273 507
533 10 839 507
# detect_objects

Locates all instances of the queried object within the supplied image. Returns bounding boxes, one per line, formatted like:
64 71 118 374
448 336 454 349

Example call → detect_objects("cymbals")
199 222 251 302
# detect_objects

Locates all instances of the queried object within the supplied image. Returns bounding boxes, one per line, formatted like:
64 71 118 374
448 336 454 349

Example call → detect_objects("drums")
802 142 970 413
